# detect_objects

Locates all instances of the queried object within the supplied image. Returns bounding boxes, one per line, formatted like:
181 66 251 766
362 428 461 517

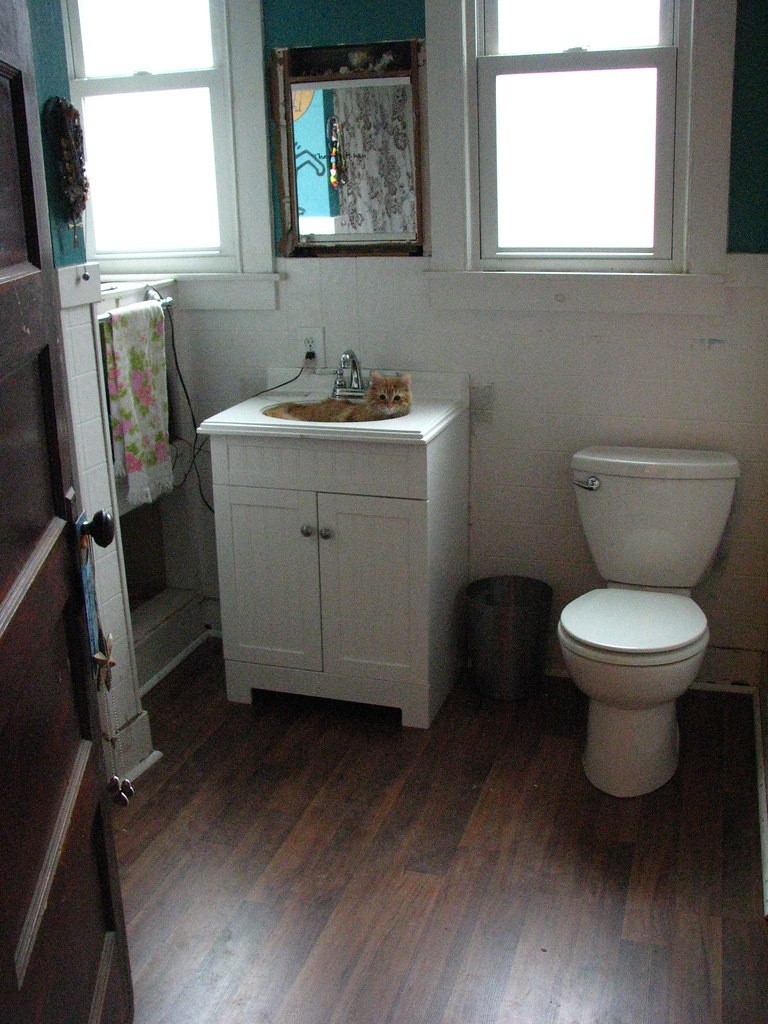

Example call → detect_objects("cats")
264 370 411 422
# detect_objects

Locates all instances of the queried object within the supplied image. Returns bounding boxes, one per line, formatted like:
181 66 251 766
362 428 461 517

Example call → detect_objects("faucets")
339 348 364 388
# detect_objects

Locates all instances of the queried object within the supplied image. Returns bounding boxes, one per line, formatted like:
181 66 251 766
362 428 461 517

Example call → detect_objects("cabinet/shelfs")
210 407 478 730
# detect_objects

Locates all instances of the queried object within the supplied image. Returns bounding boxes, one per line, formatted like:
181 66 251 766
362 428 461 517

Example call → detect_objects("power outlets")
298 326 325 368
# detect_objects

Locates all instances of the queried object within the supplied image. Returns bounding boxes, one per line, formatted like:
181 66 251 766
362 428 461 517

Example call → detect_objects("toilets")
556 441 740 799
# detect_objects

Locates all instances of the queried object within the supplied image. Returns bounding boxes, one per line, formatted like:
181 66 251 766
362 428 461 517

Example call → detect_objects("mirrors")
271 39 433 258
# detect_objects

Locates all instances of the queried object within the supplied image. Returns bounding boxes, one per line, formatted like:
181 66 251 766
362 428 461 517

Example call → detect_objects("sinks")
258 394 414 425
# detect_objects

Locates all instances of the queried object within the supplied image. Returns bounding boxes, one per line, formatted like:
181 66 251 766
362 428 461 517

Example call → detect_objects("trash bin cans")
464 575 554 705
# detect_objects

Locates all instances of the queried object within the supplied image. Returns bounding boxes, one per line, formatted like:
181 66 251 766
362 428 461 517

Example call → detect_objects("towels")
95 298 176 507
